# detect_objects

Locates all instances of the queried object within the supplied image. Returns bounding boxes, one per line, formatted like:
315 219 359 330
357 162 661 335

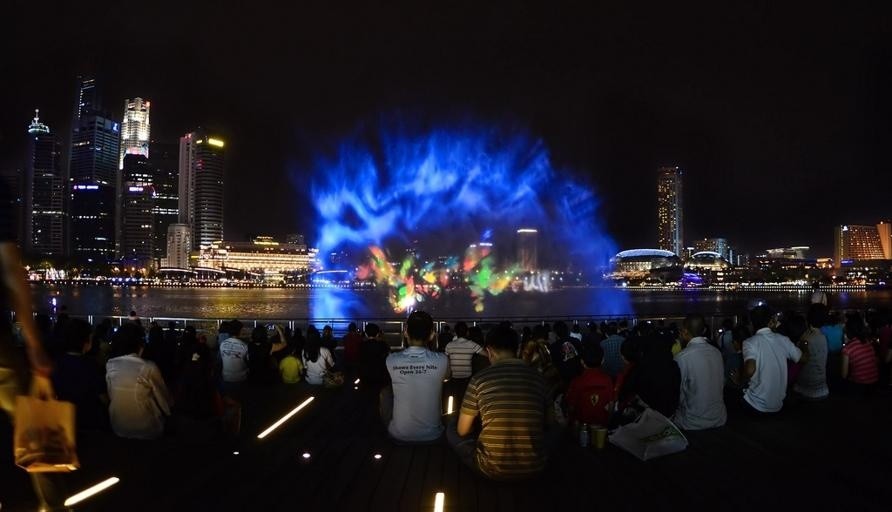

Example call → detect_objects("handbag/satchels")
611 410 688 463
14 376 81 474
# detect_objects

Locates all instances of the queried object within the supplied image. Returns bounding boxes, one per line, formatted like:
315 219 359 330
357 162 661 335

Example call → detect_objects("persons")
0 196 58 512
105 323 175 441
385 310 451 444
564 343 619 431
35 304 892 402
667 312 729 432
724 310 808 417
445 325 554 481
